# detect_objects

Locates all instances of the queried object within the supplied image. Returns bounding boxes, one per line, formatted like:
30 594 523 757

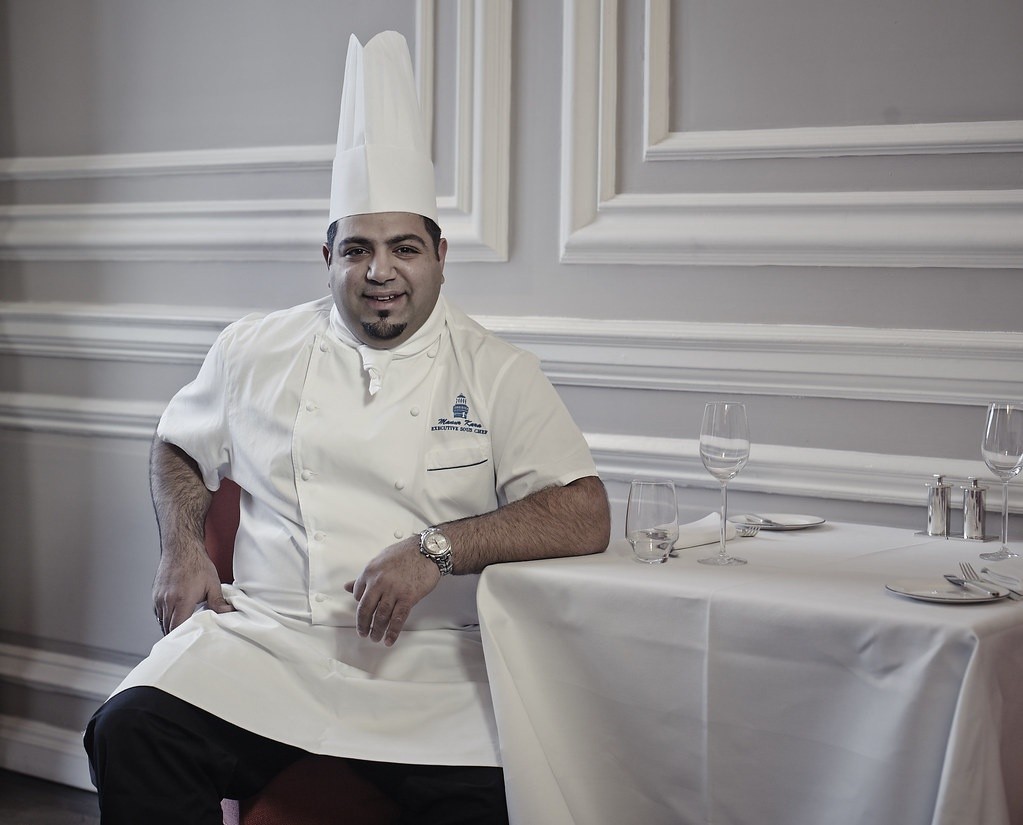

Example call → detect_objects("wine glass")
978 402 1023 560
697 400 749 567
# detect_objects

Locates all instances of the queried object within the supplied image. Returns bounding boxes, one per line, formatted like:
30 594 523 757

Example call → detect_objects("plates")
884 576 1009 603
727 513 825 532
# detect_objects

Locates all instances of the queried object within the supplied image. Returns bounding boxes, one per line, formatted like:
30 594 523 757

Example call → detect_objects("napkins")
980 553 1023 586
656 513 738 553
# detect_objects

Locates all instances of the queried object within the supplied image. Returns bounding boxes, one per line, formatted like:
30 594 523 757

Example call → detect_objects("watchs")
411 528 454 577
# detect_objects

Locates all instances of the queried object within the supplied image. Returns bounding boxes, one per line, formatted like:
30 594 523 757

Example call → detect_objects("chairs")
193 469 414 823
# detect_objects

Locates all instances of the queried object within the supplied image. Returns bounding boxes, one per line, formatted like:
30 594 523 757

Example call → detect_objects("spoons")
642 527 669 541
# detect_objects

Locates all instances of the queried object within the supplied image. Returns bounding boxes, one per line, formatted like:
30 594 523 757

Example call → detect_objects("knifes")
943 573 1001 598
745 508 786 528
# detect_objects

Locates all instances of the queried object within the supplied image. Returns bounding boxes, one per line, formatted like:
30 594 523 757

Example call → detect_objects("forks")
959 561 1022 596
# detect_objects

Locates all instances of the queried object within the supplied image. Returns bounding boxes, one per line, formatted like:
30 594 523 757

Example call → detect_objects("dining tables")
473 512 1022 824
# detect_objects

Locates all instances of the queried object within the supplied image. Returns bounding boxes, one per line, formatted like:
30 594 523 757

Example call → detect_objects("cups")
625 476 679 565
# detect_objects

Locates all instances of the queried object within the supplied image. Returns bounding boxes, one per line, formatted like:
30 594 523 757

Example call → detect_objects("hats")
328 28 445 234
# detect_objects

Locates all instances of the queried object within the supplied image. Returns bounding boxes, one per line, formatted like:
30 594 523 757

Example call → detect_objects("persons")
77 169 615 825
